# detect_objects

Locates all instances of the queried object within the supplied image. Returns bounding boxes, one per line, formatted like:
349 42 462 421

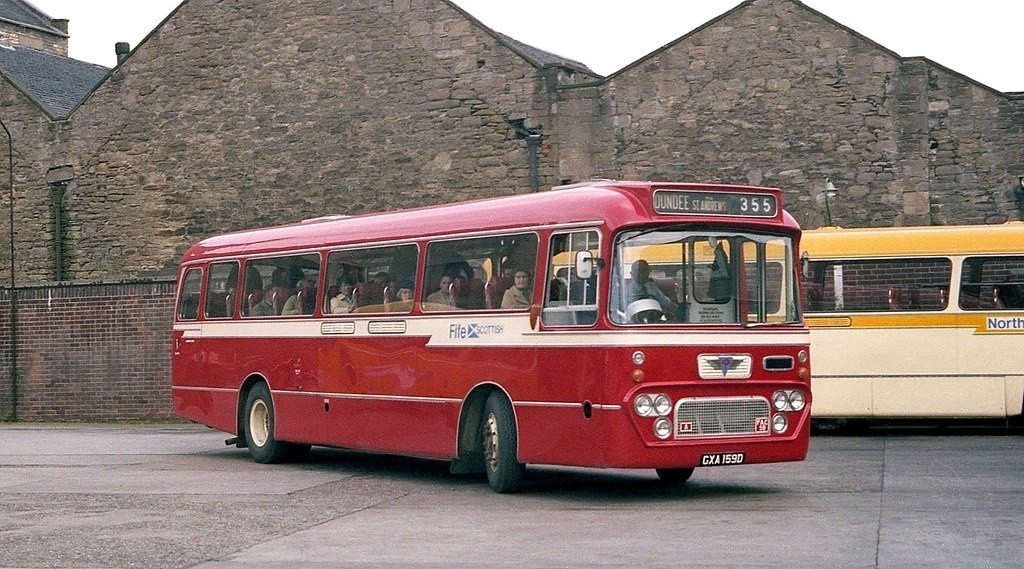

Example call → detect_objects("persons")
203 236 566 334
621 259 681 322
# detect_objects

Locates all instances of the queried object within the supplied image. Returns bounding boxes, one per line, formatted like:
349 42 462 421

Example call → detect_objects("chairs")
188 273 554 309
809 282 1024 310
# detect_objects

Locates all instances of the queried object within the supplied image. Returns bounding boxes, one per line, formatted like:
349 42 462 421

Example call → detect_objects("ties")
642 287 647 294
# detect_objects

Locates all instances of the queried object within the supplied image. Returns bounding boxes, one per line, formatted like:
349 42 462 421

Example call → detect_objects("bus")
483 218 1024 436
170 176 816 489
